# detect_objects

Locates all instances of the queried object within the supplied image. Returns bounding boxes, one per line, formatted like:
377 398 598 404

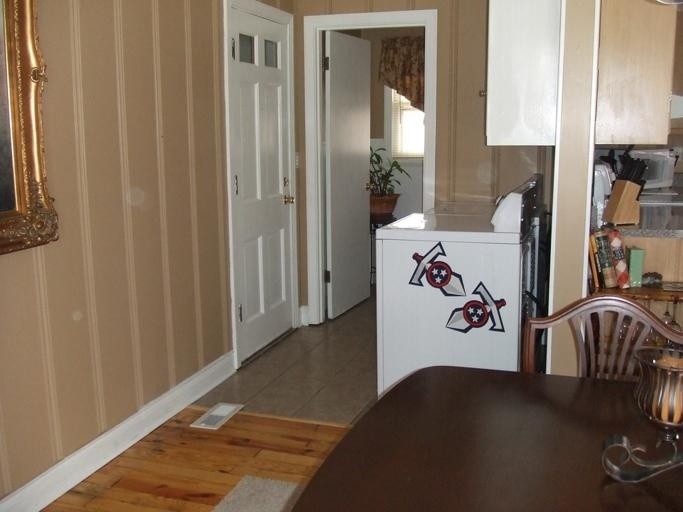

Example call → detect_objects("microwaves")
594 145 679 191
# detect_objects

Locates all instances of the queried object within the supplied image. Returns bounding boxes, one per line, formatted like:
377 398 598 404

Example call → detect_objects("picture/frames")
0 0 61 255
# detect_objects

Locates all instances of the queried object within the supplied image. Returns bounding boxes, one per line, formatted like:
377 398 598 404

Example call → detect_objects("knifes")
616 155 650 203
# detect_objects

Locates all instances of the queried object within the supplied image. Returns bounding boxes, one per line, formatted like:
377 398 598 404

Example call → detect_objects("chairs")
518 291 683 380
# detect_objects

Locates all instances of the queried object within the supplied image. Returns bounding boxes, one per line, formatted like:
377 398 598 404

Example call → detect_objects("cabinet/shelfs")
595 0 677 145
480 0 566 146
369 217 397 288
587 281 683 379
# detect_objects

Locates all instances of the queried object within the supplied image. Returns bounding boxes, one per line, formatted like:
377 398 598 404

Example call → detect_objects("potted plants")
369 146 411 217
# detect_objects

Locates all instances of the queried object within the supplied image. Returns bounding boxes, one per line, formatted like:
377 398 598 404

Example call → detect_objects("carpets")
211 475 297 512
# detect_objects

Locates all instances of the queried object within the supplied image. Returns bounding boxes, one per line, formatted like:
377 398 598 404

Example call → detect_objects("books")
589 226 645 291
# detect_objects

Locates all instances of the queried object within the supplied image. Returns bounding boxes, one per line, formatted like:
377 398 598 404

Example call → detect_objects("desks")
291 364 683 512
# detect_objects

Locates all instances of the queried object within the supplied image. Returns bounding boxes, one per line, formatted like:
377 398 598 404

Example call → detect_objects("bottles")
596 235 618 289
